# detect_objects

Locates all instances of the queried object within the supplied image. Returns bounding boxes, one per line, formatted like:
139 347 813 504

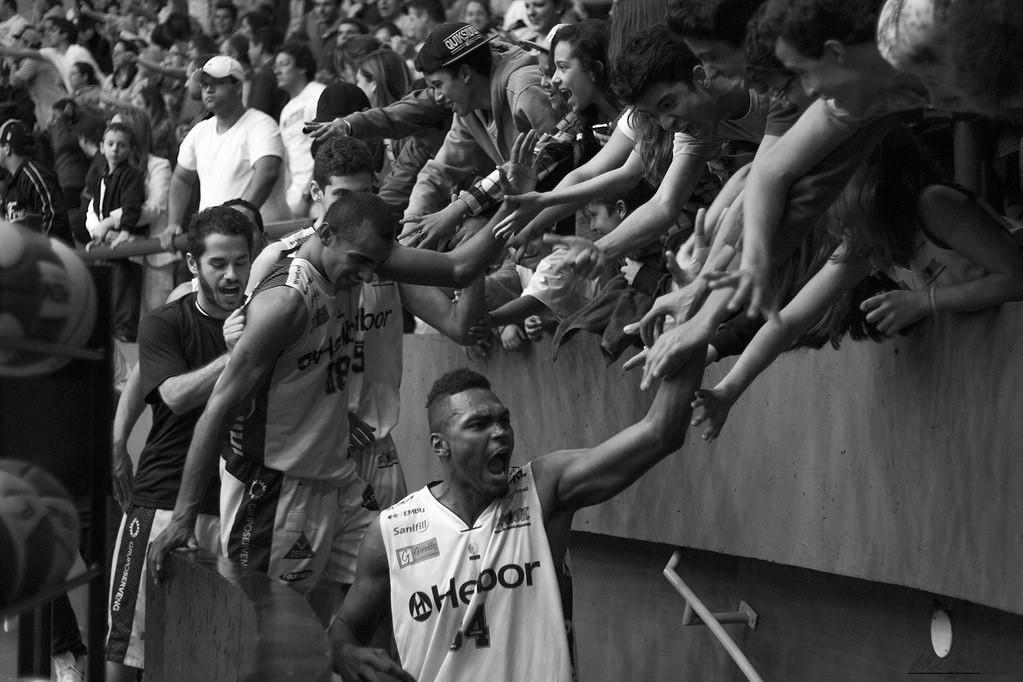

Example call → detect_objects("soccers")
1 459 82 606
1 217 100 379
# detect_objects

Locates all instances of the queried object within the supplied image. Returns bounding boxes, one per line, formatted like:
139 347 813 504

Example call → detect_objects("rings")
418 229 426 237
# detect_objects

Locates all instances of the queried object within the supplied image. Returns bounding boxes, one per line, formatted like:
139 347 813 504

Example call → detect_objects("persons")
0 0 1023 682
159 56 293 254
274 44 327 219
146 130 561 682
327 207 744 682
105 206 250 682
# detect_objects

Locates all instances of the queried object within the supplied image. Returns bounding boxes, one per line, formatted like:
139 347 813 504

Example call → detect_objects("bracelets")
930 287 940 317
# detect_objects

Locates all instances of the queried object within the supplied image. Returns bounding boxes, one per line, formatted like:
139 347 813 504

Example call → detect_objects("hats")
12 24 44 48
303 80 372 134
420 23 499 73
0 118 36 155
191 55 244 84
152 12 202 48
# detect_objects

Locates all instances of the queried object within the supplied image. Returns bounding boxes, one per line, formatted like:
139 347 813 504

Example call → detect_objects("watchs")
302 189 312 201
